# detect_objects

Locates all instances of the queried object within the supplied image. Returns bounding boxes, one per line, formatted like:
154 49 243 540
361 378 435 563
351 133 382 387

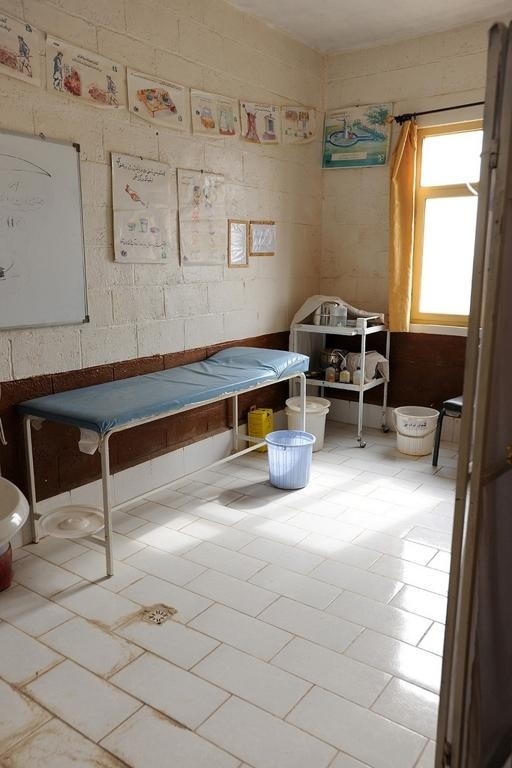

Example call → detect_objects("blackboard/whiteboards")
0 128 90 331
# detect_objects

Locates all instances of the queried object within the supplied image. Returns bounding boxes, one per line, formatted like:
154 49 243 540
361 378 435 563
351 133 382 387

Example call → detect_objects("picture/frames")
228 219 249 268
250 220 275 256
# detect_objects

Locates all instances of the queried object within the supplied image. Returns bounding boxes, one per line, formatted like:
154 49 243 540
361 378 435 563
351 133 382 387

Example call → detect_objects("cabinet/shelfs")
289 296 390 448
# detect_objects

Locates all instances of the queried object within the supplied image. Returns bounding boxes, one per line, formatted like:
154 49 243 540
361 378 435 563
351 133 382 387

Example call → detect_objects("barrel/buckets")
392 407 440 457
285 406 329 453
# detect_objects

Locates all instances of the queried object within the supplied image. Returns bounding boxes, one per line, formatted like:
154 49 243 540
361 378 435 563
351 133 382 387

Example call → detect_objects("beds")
15 346 309 576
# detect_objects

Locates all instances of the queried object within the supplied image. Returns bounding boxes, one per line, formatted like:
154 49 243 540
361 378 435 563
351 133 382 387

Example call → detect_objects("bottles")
353 367 365 384
325 353 338 381
340 366 351 383
333 303 347 325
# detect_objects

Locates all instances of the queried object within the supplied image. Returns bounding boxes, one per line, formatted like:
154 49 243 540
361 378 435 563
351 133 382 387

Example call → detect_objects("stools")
433 395 468 467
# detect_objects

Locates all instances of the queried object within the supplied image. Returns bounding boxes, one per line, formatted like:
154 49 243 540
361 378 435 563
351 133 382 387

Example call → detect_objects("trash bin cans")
265 430 315 489
